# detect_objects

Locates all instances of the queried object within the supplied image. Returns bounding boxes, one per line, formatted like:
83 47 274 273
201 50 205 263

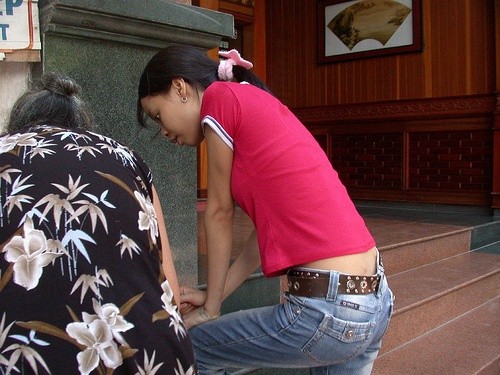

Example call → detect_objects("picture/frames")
317 0 421 63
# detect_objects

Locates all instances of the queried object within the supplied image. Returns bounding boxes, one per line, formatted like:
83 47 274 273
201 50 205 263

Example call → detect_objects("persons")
136 44 395 375
0 72 198 375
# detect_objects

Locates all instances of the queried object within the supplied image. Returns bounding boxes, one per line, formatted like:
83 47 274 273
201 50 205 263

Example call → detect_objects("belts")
287 251 382 297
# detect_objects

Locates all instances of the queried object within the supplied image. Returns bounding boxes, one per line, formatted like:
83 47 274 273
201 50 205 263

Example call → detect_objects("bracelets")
197 306 219 321
